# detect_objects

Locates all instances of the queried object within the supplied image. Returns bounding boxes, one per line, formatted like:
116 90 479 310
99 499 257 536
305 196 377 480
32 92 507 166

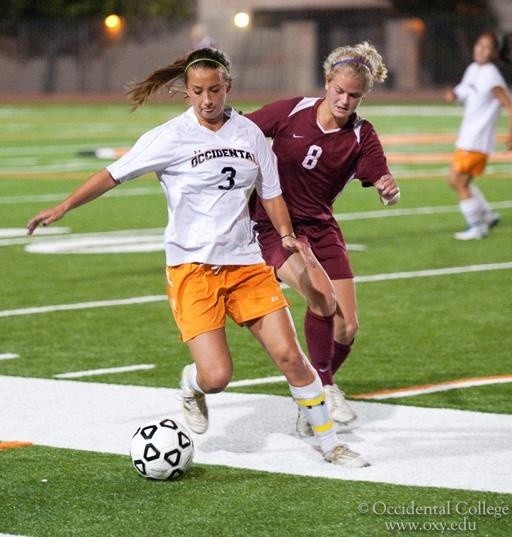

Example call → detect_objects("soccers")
130 417 194 480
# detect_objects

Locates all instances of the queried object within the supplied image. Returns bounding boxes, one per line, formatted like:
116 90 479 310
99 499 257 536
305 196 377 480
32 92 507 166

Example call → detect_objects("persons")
26 46 370 468
240 44 401 440
441 28 512 240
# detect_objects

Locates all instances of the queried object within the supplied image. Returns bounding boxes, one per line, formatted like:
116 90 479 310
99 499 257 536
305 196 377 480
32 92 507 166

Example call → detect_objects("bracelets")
281 235 296 239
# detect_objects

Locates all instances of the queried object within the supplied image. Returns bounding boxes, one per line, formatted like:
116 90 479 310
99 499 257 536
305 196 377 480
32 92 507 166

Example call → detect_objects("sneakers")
296 406 314 438
483 211 501 228
454 223 489 241
323 383 357 423
321 443 369 468
176 365 209 434
